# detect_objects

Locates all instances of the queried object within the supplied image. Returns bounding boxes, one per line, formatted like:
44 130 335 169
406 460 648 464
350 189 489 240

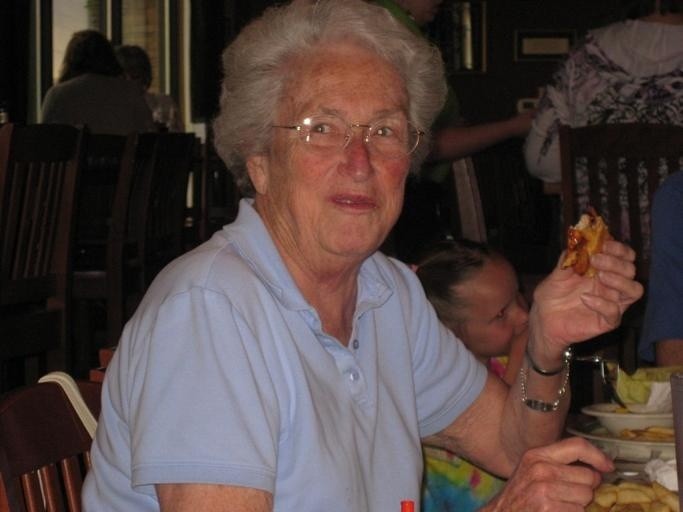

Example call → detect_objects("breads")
583 479 683 512
560 208 616 276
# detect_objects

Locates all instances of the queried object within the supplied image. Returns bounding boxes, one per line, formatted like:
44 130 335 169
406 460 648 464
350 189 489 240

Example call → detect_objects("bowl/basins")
611 380 672 413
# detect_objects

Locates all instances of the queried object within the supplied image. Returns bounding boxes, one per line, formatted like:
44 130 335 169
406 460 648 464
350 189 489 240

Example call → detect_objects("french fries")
598 425 675 443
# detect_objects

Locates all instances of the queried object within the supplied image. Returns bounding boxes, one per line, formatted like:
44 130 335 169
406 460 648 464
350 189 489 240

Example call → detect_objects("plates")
581 403 673 437
566 426 677 463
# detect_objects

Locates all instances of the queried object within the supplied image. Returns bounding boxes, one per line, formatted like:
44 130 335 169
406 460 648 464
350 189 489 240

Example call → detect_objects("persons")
368 1 542 168
638 170 683 376
43 27 154 275
387 227 529 510
522 0 681 284
116 42 184 135
79 1 642 510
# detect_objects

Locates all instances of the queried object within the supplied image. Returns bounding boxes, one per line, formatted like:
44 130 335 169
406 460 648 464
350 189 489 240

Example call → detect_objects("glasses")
277 115 425 163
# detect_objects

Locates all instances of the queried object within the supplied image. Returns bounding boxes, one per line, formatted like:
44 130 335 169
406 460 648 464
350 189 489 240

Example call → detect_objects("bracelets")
514 356 571 412
521 333 575 374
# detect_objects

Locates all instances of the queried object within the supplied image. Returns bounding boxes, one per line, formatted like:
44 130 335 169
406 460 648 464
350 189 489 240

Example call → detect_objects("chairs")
0 119 204 512
559 120 682 403
